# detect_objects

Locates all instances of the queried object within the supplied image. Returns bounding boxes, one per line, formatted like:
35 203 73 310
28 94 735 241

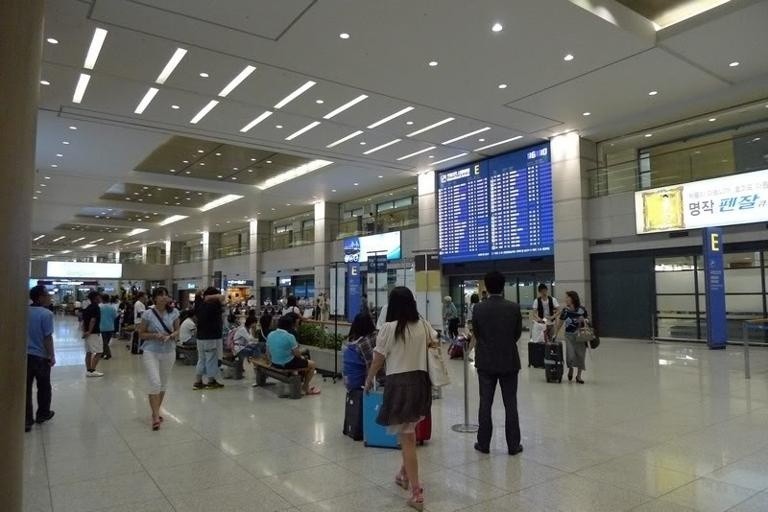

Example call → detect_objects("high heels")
406 487 425 512
576 376 584 383
394 470 409 490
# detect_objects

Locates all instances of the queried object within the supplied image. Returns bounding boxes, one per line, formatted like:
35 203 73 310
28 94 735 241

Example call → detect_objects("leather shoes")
36 411 55 424
508 444 523 456
25 419 35 432
474 442 490 454
567 368 573 381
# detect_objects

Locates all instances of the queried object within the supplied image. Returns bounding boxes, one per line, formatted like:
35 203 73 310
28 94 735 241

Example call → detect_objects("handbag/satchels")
530 319 549 344
574 323 596 343
585 322 600 349
426 342 452 387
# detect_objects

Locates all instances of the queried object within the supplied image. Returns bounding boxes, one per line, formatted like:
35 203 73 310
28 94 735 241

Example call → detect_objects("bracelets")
203 295 205 300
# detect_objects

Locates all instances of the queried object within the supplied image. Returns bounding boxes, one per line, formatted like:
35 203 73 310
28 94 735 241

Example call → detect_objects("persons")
137 286 180 431
386 214 395 231
192 286 225 390
466 293 478 361
531 284 559 344
366 212 374 235
362 286 439 512
62 282 329 376
480 291 487 302
25 285 54 432
375 214 384 233
471 269 524 455
552 290 591 384
442 296 460 340
265 315 321 395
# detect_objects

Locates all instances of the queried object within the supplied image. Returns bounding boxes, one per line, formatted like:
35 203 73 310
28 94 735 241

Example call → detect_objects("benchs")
248 357 313 401
220 352 244 379
175 342 199 366
120 327 136 340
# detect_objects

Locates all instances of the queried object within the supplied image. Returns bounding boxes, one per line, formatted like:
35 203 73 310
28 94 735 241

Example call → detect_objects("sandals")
151 414 160 429
159 416 163 422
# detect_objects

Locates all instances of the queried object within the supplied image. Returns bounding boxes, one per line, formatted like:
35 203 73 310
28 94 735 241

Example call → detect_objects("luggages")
447 336 470 359
543 340 565 383
130 328 145 354
526 339 546 369
343 388 363 441
360 385 403 449
414 407 433 446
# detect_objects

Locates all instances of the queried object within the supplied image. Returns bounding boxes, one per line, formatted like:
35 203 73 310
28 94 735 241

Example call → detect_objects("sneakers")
205 379 224 389
85 369 104 377
192 381 208 390
